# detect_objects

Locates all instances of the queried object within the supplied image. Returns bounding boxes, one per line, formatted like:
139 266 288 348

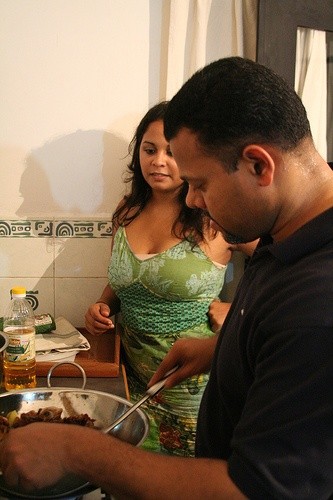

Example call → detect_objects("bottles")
0 314 56 334
3 286 37 392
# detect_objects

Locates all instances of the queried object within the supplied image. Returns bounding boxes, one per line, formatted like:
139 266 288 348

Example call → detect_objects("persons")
84 100 260 458
0 56 333 500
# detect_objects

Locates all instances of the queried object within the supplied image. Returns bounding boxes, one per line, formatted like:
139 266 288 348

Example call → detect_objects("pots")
0 362 149 498
